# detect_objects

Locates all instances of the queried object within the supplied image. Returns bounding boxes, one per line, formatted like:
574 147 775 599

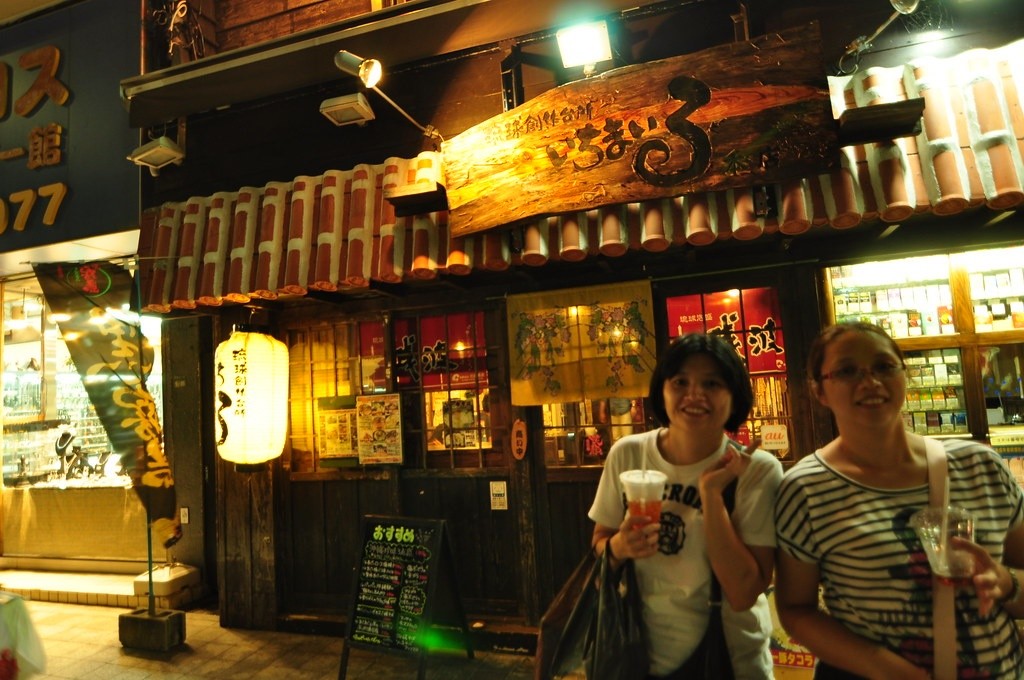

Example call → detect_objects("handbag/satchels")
535 538 651 680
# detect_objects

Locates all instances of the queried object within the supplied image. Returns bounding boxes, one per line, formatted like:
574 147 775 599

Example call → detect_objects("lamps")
556 20 614 79
126 135 184 169
838 0 920 75
334 50 439 139
319 92 376 127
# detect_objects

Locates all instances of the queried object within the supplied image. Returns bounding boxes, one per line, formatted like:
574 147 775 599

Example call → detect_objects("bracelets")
1000 568 1020 605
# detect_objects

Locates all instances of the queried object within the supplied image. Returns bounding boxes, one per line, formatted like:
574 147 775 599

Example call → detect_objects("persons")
587 333 785 680
775 322 1024 680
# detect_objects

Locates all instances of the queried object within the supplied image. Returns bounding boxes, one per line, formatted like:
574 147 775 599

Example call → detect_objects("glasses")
815 358 908 383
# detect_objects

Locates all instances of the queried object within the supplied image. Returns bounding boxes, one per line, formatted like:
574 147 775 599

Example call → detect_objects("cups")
620 469 666 546
910 506 975 576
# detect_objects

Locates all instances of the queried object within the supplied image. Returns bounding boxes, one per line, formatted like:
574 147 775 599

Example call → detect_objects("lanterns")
213 324 289 472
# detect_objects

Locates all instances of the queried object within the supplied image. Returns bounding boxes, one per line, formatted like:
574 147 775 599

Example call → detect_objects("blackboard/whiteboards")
346 515 470 657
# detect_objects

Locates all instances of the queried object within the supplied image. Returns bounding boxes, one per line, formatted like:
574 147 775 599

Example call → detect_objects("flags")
29 260 182 548
